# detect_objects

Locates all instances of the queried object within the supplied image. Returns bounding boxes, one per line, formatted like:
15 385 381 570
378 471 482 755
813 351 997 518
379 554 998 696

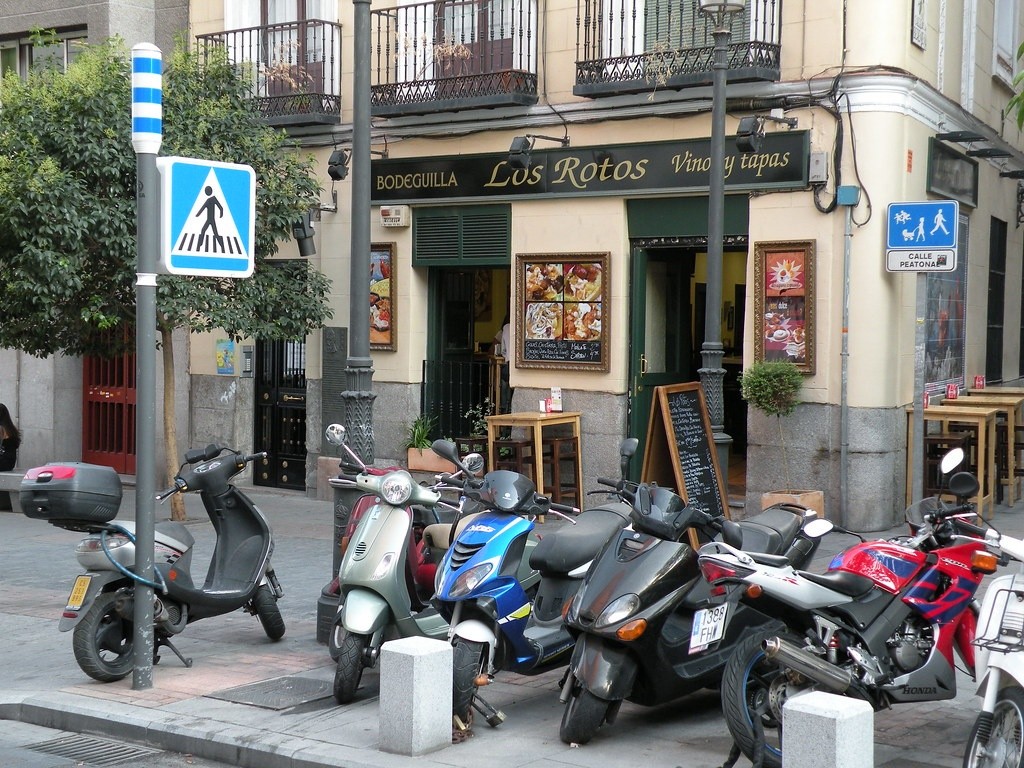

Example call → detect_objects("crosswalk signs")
161 153 257 280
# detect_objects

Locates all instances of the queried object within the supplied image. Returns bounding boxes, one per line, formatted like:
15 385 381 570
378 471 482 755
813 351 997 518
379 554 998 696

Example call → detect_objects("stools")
493 436 581 521
924 410 1024 516
456 436 488 501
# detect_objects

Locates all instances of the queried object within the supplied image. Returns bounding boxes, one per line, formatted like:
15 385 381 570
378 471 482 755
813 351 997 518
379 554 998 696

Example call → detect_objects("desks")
941 396 1024 507
484 412 584 524
906 406 999 526
487 354 508 435
968 387 1024 397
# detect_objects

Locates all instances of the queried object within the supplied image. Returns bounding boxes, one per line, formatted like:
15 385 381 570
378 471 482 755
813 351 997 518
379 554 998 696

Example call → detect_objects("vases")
408 448 509 477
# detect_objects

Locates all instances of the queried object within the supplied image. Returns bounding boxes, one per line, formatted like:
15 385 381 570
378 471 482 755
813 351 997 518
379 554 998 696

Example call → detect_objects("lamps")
936 131 988 143
735 115 798 154
966 148 1014 158
328 148 388 180
508 134 570 170
291 190 338 256
1001 170 1024 178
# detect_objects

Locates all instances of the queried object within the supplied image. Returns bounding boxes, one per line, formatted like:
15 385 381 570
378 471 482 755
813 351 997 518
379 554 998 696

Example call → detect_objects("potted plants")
737 357 825 523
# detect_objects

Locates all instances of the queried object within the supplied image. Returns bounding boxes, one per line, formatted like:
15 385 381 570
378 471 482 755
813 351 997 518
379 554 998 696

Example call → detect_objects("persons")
488 313 514 406
0 403 22 514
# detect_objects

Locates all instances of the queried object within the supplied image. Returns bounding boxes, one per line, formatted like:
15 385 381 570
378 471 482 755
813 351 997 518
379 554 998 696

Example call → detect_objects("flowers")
398 398 513 461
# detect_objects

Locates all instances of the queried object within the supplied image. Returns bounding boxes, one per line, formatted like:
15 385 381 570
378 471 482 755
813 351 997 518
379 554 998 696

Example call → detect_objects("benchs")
0 468 26 513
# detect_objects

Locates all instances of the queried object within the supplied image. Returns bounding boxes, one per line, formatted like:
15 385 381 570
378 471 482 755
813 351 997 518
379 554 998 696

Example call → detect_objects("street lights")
698 0 748 520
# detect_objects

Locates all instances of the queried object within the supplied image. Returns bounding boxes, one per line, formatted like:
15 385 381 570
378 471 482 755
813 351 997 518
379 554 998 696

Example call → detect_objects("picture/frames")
369 242 398 352
515 252 610 373
475 270 492 321
753 239 817 376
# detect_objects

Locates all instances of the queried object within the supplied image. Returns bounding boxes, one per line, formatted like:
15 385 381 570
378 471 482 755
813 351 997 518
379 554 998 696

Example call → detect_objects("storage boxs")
19 462 122 523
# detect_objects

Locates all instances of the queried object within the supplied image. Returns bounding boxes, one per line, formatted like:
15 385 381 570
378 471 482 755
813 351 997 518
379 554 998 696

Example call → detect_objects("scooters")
430 438 635 729
555 436 824 747
324 423 490 706
18 442 287 682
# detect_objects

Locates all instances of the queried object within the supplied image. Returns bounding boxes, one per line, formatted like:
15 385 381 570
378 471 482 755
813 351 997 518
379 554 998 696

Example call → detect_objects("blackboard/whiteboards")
641 381 732 548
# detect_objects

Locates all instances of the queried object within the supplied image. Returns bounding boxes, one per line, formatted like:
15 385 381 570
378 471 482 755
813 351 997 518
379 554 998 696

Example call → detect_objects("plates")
763 313 805 344
777 271 791 283
370 291 392 332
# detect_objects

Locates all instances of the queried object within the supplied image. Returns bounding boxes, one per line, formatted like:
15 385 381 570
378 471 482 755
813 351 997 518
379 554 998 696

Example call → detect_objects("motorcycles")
695 450 997 768
946 470 1023 768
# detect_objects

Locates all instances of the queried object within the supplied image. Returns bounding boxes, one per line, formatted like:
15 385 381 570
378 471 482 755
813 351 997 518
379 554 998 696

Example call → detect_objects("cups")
779 269 788 280
